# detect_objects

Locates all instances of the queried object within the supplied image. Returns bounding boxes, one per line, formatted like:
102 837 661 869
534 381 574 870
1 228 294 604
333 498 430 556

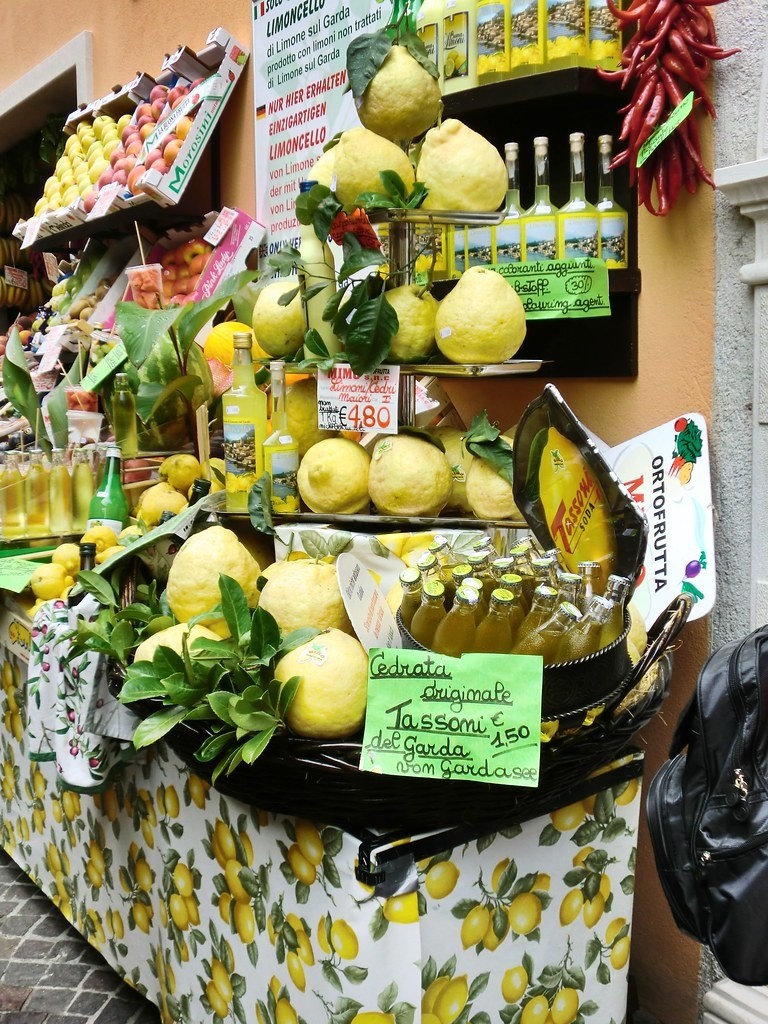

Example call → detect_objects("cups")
125 263 165 310
65 386 99 412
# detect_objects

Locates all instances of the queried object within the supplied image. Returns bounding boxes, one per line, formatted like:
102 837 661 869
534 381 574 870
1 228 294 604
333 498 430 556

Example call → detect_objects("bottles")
451 130 630 281
394 536 632 663
261 359 302 516
0 445 127 610
222 332 268 514
188 478 212 508
111 373 139 460
158 510 177 526
416 0 622 93
299 180 342 361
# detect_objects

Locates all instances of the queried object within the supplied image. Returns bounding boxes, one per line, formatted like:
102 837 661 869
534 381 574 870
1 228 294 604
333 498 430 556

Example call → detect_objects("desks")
0 595 644 1024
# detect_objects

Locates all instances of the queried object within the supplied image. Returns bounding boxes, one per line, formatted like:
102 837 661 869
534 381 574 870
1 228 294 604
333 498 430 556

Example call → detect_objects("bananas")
0 192 57 312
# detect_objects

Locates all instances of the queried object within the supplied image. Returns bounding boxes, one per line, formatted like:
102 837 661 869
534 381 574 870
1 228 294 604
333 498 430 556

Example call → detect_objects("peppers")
596 0 744 215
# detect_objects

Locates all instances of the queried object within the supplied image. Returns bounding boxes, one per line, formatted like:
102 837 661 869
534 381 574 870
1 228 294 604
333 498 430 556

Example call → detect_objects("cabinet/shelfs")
413 0 640 382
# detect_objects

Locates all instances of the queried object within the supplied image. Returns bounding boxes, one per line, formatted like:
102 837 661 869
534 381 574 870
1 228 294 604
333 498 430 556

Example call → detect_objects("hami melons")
0 460 92 539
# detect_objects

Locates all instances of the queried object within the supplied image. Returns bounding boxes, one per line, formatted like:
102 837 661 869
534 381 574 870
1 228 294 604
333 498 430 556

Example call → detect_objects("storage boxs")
32 206 267 354
13 26 250 239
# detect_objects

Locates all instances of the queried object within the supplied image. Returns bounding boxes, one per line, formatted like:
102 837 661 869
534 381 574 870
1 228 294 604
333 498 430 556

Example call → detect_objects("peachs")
0 311 38 354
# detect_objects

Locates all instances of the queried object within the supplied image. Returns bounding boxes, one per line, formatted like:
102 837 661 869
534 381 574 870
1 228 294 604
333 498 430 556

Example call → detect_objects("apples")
30 75 206 212
156 235 215 308
121 457 151 483
32 253 101 331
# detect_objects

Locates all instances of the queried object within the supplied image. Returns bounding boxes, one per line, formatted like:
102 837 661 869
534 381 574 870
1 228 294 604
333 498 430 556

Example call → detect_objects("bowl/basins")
42 409 107 444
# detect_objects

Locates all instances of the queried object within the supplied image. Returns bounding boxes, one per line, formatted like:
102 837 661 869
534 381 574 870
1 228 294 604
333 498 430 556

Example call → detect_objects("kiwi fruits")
62 275 116 325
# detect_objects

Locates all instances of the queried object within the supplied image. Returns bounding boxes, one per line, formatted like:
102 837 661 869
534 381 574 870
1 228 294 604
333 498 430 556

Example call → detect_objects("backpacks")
645 621 768 987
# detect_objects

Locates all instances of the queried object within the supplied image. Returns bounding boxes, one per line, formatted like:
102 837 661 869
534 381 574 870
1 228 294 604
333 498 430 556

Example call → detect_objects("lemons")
274 375 525 523
252 44 528 364
202 321 264 370
539 427 616 586
1 659 639 1024
26 454 224 620
132 526 373 737
